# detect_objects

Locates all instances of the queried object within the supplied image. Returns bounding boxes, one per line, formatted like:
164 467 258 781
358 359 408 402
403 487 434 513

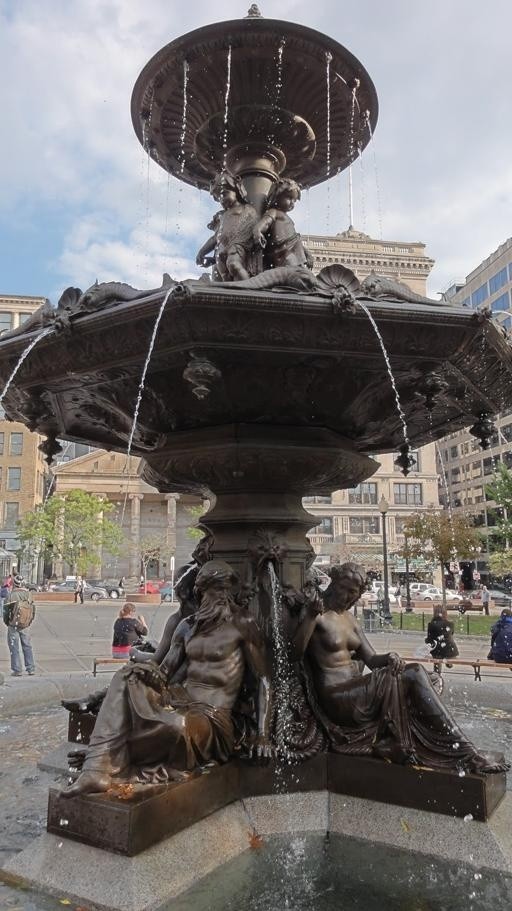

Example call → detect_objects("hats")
13 574 25 588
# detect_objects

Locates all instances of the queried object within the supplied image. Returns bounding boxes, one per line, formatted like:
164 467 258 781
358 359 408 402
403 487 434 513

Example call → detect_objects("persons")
393 584 401 602
256 179 311 269
112 603 151 660
119 577 126 595
425 603 460 676
4 574 39 677
72 574 85 605
377 585 384 607
63 563 279 801
3 575 12 588
295 557 512 773
13 571 24 588
60 563 202 720
489 608 511 664
195 176 256 278
482 586 490 616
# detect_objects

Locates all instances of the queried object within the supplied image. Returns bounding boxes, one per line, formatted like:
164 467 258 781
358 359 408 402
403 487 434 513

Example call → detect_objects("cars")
357 579 512 613
13 574 180 604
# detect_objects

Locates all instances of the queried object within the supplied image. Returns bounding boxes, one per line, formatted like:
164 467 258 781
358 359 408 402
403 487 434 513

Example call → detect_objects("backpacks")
8 592 36 630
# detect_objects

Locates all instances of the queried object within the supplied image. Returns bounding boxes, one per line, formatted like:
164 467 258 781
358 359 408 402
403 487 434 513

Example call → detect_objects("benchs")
445 602 483 612
91 654 141 679
393 646 512 680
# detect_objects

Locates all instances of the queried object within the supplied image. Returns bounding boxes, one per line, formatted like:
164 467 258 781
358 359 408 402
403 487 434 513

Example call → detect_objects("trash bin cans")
362 601 379 632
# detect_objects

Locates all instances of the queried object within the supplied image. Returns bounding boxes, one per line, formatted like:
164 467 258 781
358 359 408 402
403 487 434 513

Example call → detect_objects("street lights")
377 493 394 625
400 527 412 613
69 542 82 562
